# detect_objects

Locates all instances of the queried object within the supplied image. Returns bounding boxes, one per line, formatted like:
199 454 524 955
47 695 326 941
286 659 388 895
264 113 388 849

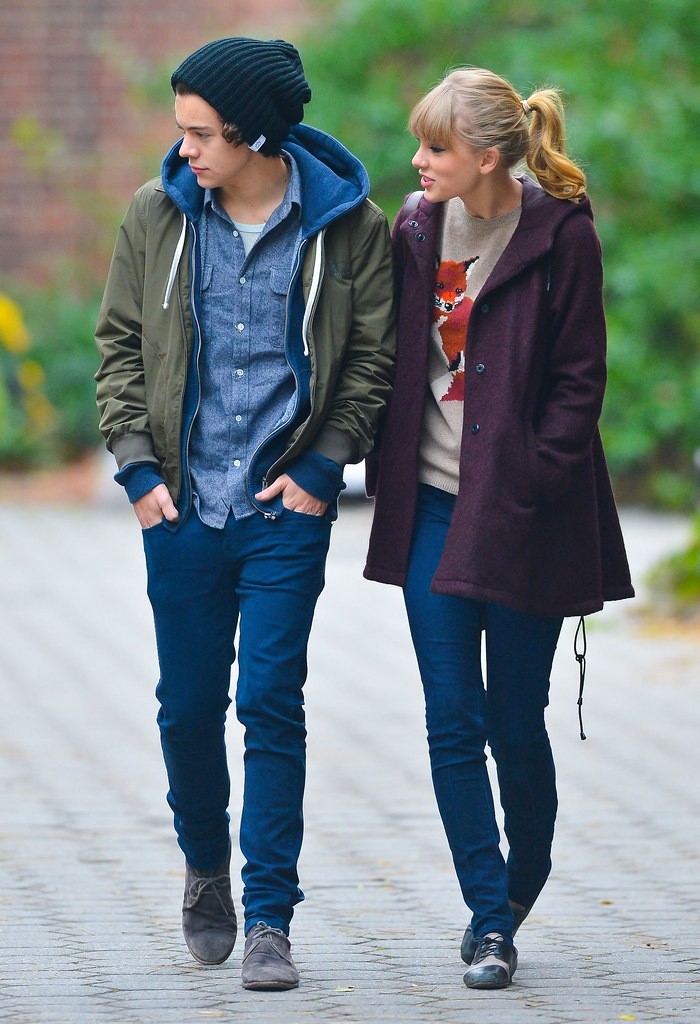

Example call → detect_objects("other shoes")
182 835 237 965
242 921 299 991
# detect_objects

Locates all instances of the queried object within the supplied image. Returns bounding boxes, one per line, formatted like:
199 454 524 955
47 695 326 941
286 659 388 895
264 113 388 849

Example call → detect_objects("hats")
171 37 312 156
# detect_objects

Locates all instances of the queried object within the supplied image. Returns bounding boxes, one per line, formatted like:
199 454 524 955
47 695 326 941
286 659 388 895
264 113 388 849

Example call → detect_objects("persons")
361 66 640 993
92 31 397 990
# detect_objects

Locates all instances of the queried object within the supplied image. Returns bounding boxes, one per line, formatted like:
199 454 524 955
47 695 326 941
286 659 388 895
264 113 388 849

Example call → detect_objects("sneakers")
460 860 534 965
463 936 518 989
